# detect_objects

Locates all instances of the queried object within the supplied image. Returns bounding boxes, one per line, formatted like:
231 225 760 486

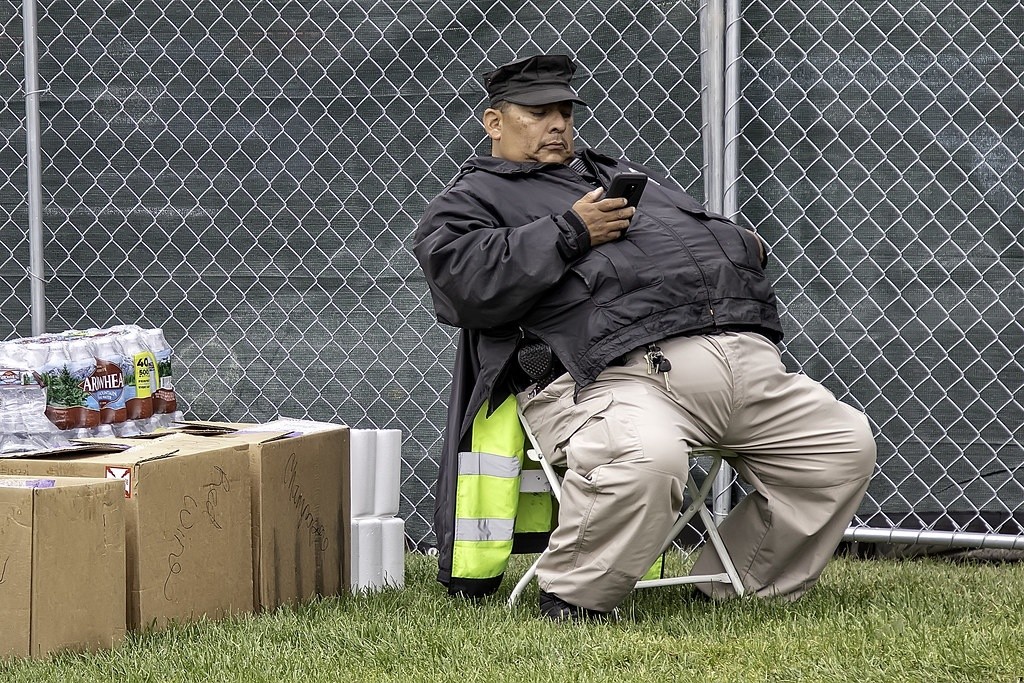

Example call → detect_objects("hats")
481 54 587 107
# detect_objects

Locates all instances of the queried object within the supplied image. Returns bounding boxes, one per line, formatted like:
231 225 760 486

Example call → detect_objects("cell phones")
602 173 649 242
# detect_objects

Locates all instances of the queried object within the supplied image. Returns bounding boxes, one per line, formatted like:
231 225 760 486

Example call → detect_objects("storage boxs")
0 419 352 668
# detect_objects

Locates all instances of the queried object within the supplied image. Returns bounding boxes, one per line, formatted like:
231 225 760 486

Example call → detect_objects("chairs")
505 397 753 620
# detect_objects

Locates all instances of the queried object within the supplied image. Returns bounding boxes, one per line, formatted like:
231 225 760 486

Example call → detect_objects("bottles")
0 325 178 454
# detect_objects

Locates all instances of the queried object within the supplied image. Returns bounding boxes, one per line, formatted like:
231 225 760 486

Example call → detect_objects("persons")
413 54 876 623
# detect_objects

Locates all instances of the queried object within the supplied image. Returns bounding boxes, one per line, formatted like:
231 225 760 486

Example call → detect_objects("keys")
643 343 673 393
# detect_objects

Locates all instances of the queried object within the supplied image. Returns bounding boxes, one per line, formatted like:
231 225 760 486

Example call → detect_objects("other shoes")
538 587 602 625
694 588 713 604
